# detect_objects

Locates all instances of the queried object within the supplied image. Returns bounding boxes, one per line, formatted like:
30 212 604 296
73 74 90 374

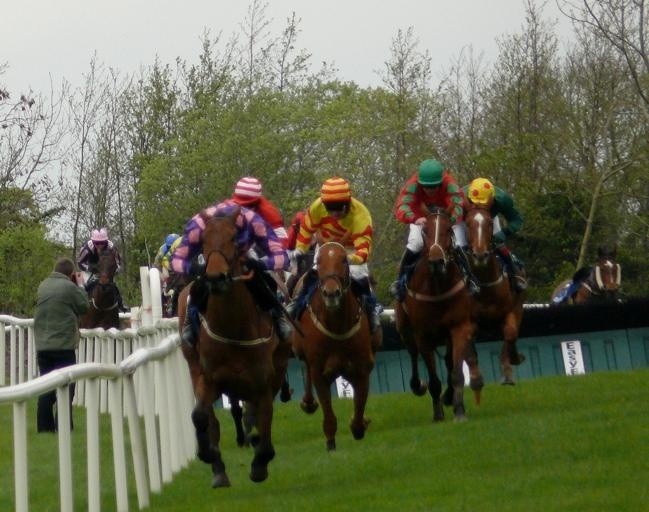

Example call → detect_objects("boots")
453 245 481 289
393 247 423 303
283 268 320 320
496 244 520 291
266 281 292 345
115 287 128 312
180 285 204 348
351 277 379 334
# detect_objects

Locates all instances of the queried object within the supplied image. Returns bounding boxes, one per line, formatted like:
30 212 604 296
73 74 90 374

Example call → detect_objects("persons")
153 175 387 357
78 228 131 314
388 160 526 301
33 259 89 433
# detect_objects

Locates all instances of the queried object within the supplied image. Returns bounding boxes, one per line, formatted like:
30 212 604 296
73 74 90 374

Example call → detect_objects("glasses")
324 202 345 212
93 241 105 245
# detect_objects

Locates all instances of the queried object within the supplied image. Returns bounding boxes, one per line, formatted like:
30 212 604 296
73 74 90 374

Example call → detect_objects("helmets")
468 176 496 207
320 175 352 203
165 233 184 249
211 207 250 246
231 176 263 206
415 158 445 187
90 227 109 241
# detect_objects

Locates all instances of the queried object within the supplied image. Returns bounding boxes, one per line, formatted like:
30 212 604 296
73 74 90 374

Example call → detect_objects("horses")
395 201 475 424
463 196 525 390
287 228 375 454
173 207 287 490
549 246 621 305
79 246 119 329
